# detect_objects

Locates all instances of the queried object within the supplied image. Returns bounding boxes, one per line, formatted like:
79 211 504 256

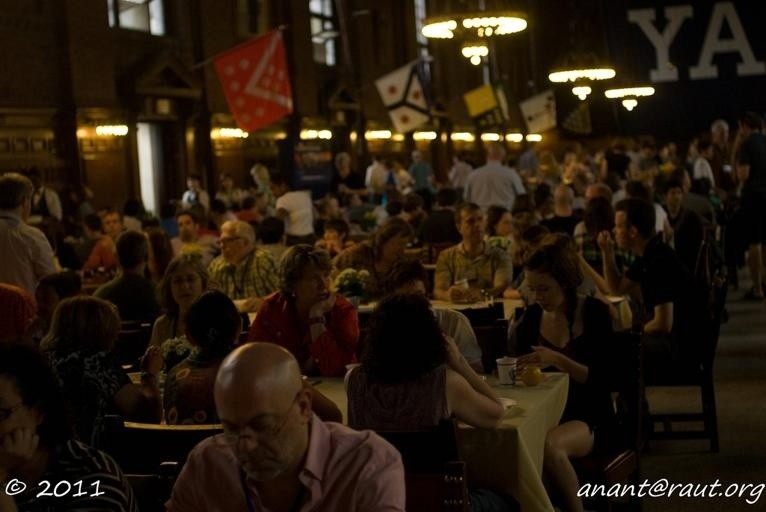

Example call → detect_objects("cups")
496 357 517 385
454 278 469 292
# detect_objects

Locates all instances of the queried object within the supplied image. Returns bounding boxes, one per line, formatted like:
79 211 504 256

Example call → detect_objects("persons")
618 182 674 244
36 296 164 469
82 213 126 277
710 117 740 284
26 167 62 247
408 151 445 198
0 172 63 331
119 197 144 236
656 185 706 249
329 218 415 293
164 289 340 465
417 189 459 243
209 220 280 314
539 186 584 234
65 181 98 265
315 225 350 256
464 140 526 207
318 183 405 221
385 261 485 383
596 199 713 445
247 243 360 378
571 182 614 241
504 233 612 296
365 156 388 190
448 152 475 188
161 342 406 512
168 213 218 262
216 175 249 211
180 175 209 213
507 247 618 512
345 292 506 511
390 159 417 197
328 153 372 204
574 201 638 272
89 230 156 321
736 110 766 300
141 249 243 374
250 163 277 214
434 201 513 302
267 175 315 245
2 284 40 347
34 272 82 327
0 345 136 512
483 207 515 242
531 133 717 182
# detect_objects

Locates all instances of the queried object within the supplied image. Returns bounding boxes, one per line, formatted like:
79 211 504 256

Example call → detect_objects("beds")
119 360 572 512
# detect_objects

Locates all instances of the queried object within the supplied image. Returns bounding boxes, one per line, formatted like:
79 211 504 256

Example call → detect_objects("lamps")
422 0 529 69
548 0 614 101
605 0 656 111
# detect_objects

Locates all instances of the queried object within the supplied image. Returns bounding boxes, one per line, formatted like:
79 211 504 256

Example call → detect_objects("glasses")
216 236 242 246
0 401 28 421
169 274 201 284
213 380 325 448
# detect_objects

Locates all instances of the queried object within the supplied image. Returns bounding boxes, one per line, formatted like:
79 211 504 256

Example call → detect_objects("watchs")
306 316 330 326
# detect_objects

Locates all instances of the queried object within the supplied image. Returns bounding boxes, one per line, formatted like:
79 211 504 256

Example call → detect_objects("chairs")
126 460 175 512
423 416 469 510
640 284 731 458
